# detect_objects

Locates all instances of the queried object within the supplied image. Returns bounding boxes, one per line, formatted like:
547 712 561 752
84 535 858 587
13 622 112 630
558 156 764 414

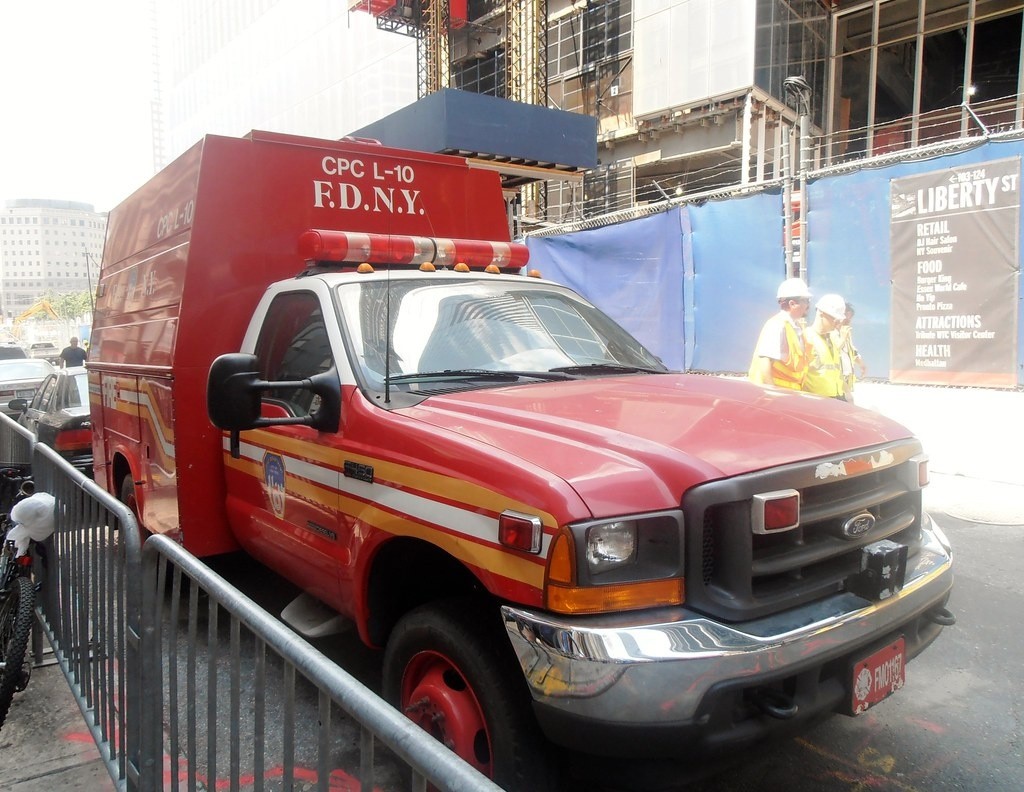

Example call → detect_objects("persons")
748 278 811 391
805 294 846 402
832 305 866 406
59 337 88 370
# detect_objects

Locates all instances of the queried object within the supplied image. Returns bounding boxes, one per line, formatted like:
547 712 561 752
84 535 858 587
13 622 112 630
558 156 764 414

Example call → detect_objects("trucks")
86 126 956 792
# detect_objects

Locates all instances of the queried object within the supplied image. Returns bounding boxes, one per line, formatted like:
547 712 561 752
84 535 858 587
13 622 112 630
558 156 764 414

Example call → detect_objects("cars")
0 342 29 359
7 366 94 480
0 359 56 481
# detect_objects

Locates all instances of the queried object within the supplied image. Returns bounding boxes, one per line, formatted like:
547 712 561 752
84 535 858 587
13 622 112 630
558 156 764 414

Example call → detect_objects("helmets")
777 277 814 300
816 293 847 322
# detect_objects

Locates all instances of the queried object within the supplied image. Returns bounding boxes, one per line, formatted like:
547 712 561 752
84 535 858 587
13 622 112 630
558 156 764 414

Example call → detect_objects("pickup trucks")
26 342 60 365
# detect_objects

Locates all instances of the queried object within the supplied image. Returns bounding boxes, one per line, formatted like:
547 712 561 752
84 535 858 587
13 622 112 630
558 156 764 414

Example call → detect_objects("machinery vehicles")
0 300 63 341
